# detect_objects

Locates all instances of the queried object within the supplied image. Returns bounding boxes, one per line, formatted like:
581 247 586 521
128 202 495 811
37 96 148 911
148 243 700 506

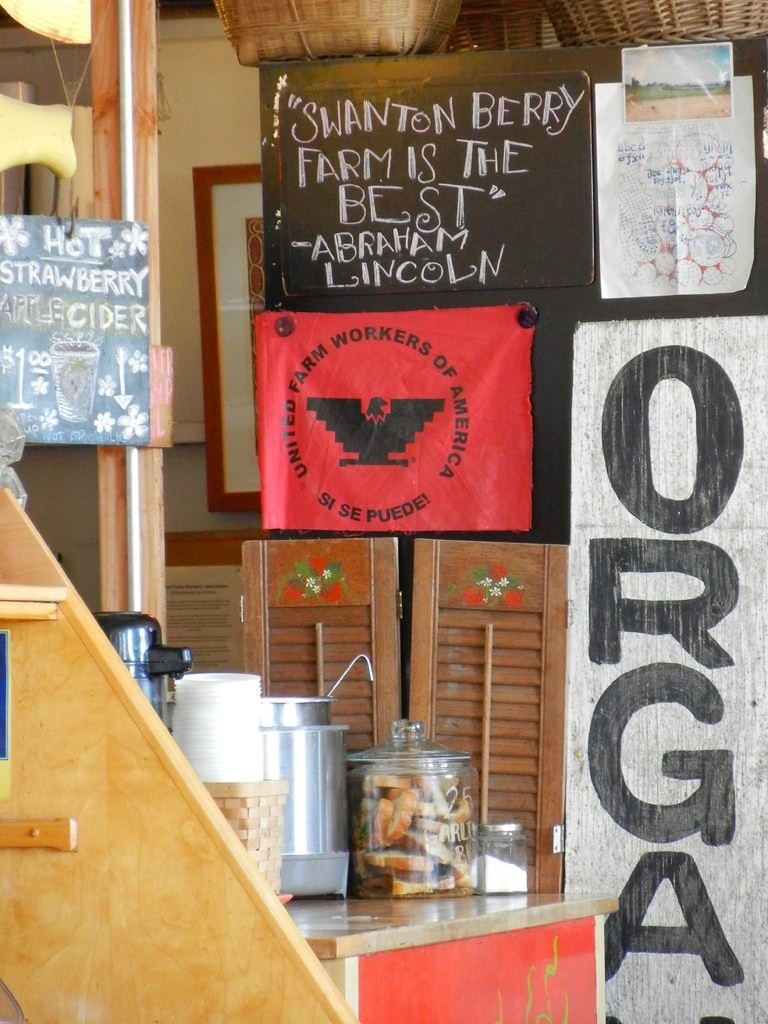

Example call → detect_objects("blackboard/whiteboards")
279 71 596 294
0 216 151 447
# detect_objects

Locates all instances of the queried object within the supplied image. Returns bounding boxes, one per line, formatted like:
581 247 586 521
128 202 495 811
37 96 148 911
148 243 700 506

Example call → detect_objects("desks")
283 891 622 1023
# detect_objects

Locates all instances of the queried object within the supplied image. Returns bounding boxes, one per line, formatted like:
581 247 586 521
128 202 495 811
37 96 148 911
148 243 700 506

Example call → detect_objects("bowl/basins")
172 672 263 785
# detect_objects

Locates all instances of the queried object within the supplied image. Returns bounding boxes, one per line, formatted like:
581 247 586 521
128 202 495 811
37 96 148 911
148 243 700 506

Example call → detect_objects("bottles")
478 823 530 895
344 719 481 900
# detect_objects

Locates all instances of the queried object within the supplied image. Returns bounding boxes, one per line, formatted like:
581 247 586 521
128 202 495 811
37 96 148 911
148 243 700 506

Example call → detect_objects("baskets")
215 0 463 67
446 0 570 53
542 0 768 47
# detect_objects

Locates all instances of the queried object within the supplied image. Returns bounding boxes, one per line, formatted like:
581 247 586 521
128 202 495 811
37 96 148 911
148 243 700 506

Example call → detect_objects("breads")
347 766 472 900
